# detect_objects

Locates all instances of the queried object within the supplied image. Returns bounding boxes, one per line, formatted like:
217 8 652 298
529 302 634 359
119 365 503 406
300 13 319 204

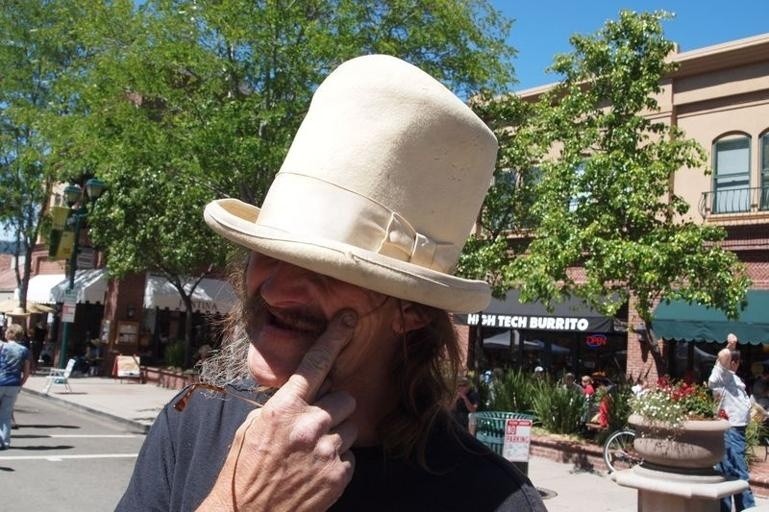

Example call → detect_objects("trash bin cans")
470 411 533 476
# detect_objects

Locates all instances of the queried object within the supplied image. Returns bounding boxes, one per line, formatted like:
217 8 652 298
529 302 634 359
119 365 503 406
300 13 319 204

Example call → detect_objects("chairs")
43 359 78 397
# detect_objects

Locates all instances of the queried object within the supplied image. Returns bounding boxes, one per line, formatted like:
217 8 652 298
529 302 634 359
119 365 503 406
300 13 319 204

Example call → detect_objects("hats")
202 52 501 318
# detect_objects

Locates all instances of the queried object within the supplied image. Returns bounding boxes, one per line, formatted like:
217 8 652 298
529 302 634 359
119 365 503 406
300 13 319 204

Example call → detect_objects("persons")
467 347 621 428
654 348 712 395
736 352 769 450
112 53 551 511
139 312 222 370
0 323 31 452
707 334 756 511
0 317 45 377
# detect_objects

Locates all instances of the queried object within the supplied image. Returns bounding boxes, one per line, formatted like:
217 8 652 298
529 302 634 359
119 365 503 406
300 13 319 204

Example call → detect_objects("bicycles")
602 425 644 473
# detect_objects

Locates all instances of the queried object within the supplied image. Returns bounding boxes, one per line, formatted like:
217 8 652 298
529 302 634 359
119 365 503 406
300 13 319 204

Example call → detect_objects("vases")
623 415 732 477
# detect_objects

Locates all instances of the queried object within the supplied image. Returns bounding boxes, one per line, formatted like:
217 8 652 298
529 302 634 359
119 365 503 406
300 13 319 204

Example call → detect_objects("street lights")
58 178 103 383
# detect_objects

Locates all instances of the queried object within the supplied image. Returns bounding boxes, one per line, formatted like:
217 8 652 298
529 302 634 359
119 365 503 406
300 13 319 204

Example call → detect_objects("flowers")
628 374 726 452
